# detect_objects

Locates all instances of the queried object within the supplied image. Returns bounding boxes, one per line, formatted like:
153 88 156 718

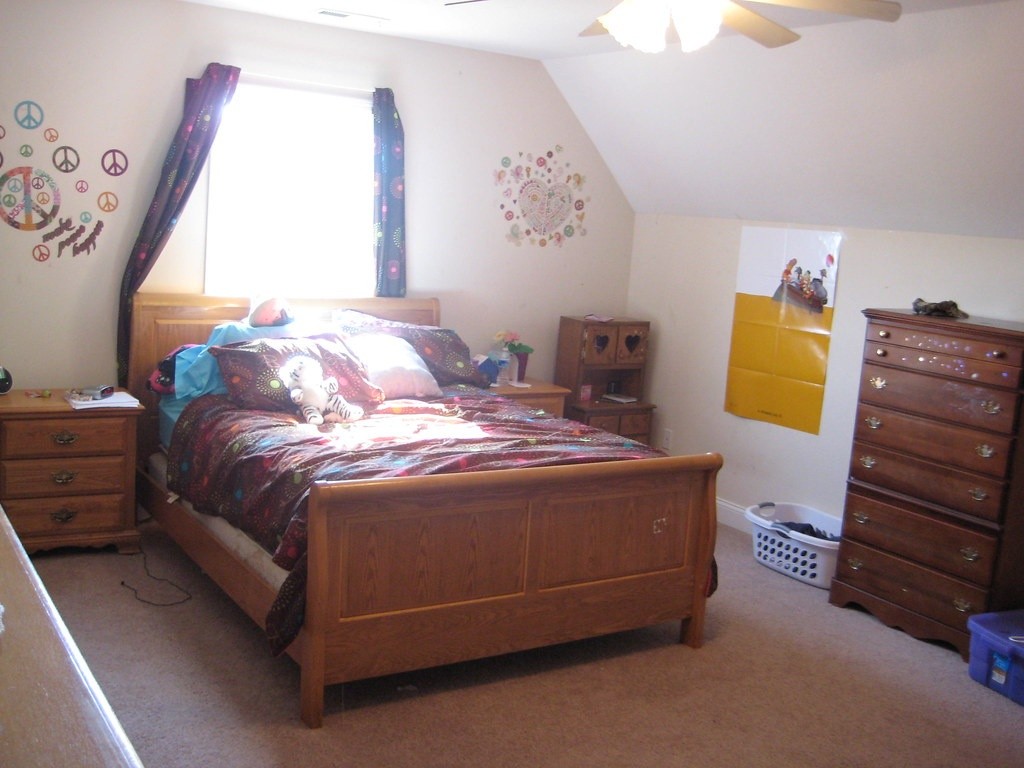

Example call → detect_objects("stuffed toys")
278 355 362 425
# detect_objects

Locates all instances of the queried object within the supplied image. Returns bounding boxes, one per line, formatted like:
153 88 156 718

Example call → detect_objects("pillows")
341 310 484 388
342 333 446 401
204 331 386 413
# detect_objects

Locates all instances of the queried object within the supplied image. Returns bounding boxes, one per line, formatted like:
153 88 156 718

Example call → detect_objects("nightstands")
480 376 572 419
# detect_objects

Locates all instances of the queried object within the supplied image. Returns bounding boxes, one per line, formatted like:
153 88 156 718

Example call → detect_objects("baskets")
745 501 843 589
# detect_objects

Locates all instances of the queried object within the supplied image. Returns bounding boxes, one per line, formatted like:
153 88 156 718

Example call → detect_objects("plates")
508 382 532 388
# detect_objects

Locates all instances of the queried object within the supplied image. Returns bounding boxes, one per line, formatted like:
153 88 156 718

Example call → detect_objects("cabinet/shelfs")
826 305 1024 663
0 386 147 559
554 314 657 445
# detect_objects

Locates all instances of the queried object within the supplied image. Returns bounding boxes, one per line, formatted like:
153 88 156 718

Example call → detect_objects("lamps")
599 0 728 53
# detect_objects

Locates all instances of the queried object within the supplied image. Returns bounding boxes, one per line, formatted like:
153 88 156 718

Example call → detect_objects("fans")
578 0 902 50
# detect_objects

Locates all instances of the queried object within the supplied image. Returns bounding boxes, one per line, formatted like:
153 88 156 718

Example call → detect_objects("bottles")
496 347 510 387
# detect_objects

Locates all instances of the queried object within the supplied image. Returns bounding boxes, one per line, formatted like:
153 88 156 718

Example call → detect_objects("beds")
131 287 723 729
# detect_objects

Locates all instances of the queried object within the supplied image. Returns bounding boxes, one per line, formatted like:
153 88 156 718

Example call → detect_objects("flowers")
494 330 520 349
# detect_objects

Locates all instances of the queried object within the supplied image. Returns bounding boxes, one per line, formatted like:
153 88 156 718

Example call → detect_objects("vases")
501 347 518 381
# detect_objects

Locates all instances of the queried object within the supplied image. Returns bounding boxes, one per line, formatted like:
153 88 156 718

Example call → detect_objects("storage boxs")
744 501 844 589
967 608 1024 709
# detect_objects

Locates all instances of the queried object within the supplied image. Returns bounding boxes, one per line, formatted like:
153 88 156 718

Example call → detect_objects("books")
602 393 637 403
